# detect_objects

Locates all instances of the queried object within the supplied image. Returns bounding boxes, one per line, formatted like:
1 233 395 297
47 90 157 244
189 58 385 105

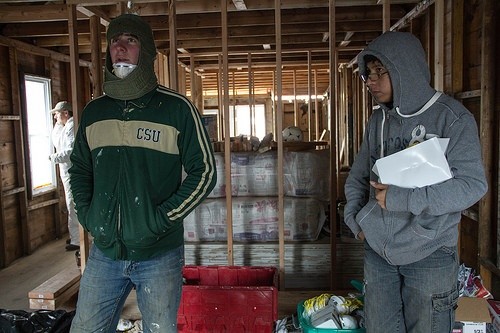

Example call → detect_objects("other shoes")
65 244 80 251
65 238 71 244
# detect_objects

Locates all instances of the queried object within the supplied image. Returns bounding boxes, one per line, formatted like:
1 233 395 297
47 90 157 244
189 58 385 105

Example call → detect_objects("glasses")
359 71 388 82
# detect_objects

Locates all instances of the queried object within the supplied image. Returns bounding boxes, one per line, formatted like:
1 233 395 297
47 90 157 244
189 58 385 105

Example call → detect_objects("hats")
102 14 159 100
49 101 73 114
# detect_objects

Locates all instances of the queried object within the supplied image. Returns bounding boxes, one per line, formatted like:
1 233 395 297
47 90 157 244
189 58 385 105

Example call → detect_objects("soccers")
282 126 304 143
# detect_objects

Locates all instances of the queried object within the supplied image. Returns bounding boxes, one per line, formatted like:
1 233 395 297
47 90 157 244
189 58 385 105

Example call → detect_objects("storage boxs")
455 297 500 333
297 300 366 333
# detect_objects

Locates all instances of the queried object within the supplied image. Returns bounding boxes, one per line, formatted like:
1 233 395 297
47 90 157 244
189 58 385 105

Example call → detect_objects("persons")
344 32 489 333
68 14 216 333
47 100 81 251
